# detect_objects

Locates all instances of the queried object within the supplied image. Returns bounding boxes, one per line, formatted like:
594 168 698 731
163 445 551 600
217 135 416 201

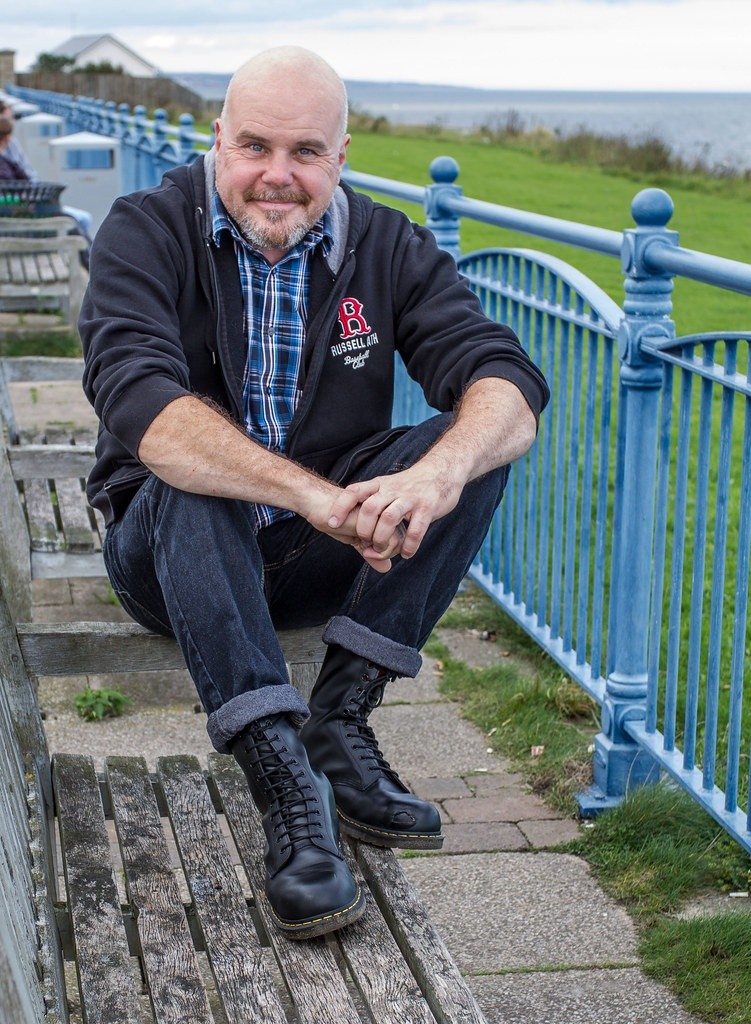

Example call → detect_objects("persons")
78 48 550 941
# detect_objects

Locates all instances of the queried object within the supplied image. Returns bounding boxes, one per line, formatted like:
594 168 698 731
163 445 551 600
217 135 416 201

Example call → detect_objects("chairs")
0 215 90 342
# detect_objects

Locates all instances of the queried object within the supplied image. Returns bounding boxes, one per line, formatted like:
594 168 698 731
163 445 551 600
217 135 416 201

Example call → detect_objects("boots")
229 715 366 938
300 644 443 851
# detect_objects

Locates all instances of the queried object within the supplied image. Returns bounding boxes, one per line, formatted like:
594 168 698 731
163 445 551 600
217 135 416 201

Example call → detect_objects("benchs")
0 581 489 1024
0 355 109 623
